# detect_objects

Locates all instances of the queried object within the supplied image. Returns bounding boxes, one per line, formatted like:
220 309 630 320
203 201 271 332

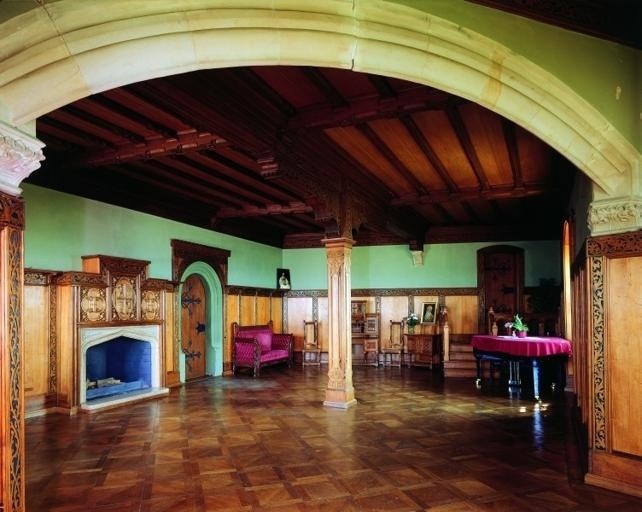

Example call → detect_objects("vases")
408 323 414 333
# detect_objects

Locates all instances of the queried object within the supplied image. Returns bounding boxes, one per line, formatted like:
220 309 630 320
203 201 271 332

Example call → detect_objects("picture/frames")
420 302 437 325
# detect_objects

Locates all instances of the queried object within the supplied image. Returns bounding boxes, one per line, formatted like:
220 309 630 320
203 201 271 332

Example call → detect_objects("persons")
279 272 290 289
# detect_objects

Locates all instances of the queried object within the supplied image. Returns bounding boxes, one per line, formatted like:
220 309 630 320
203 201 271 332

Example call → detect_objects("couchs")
231 320 294 379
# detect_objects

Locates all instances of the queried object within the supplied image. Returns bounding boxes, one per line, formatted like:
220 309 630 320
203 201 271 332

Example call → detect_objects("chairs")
302 319 321 366
381 319 405 372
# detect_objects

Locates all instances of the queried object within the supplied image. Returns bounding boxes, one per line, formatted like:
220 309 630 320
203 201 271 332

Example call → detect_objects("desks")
351 334 380 365
472 336 572 401
405 333 442 370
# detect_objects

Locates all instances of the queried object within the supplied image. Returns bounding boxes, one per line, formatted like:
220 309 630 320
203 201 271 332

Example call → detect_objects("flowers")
405 313 420 327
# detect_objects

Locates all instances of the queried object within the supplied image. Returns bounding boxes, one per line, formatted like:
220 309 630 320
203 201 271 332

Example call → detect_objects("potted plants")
512 314 530 338
